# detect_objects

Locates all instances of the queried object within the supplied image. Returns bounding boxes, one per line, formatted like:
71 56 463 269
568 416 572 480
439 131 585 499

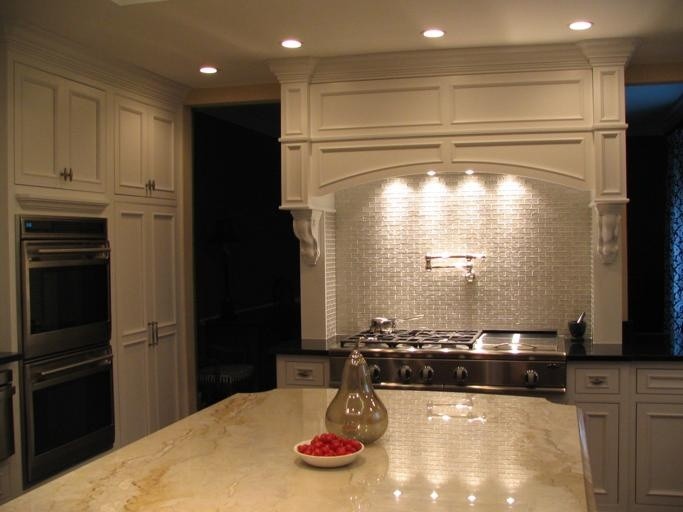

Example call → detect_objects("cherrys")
297 433 362 457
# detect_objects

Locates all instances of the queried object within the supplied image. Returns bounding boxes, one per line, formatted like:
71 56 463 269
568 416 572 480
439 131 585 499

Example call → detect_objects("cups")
568 321 585 337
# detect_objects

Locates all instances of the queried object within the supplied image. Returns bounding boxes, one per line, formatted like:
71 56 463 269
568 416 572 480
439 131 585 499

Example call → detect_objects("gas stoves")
338 328 483 388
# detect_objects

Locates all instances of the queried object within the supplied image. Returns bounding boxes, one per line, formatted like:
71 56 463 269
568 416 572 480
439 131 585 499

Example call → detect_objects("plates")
294 438 364 468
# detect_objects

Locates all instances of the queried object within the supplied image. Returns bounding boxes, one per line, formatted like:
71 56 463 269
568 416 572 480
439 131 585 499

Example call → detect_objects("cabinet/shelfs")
273 354 329 393
0 48 104 198
103 193 192 454
106 84 184 208
565 353 683 511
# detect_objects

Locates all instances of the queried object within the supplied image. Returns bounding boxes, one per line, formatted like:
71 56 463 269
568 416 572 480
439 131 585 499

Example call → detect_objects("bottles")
323 351 388 445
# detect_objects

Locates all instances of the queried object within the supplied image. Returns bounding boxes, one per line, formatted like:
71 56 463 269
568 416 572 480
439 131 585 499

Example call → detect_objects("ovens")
21 214 116 489
